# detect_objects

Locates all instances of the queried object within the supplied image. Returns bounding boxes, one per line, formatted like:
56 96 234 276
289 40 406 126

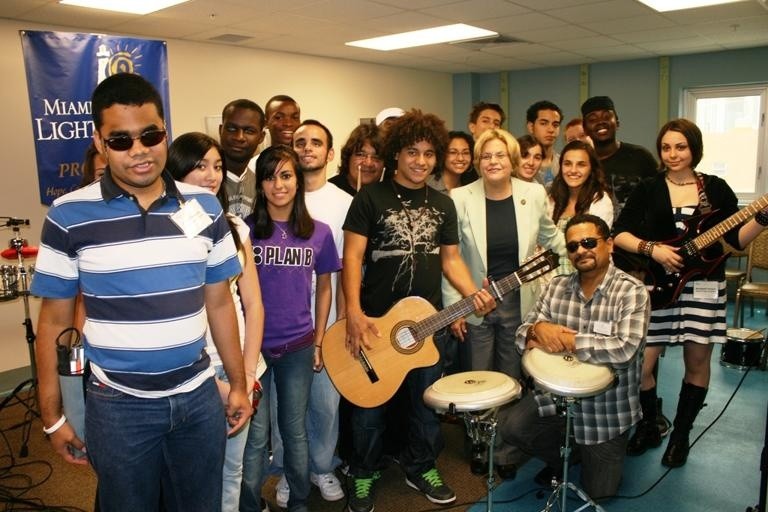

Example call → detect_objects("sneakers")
348 474 375 512
310 470 344 502
404 466 457 505
275 471 291 508
533 454 575 486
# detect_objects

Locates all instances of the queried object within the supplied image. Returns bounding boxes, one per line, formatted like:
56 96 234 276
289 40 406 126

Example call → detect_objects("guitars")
612 191 768 311
320 248 560 408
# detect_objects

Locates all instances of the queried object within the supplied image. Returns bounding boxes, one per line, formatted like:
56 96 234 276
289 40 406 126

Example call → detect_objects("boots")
660 380 708 468
626 383 662 457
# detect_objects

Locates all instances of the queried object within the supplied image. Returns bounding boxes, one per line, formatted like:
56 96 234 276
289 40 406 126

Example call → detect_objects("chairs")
723 241 751 318
729 228 767 331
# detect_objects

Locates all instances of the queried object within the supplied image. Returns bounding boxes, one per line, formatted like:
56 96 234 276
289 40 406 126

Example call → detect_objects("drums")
720 328 764 370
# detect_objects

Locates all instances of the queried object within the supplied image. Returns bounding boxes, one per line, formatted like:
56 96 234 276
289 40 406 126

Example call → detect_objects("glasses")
563 237 608 253
98 130 167 151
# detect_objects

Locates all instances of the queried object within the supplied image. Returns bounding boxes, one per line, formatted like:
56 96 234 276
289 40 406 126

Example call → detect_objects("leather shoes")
495 464 518 482
472 462 490 474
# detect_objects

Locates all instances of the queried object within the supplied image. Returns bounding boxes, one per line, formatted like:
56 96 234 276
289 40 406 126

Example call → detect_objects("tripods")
539 407 607 511
0 296 39 458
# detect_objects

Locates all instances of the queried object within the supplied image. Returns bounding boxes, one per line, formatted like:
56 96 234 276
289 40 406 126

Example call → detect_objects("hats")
581 96 615 114
375 107 406 125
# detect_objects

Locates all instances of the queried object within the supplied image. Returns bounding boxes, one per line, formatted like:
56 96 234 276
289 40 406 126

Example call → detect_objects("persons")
29 71 768 512
